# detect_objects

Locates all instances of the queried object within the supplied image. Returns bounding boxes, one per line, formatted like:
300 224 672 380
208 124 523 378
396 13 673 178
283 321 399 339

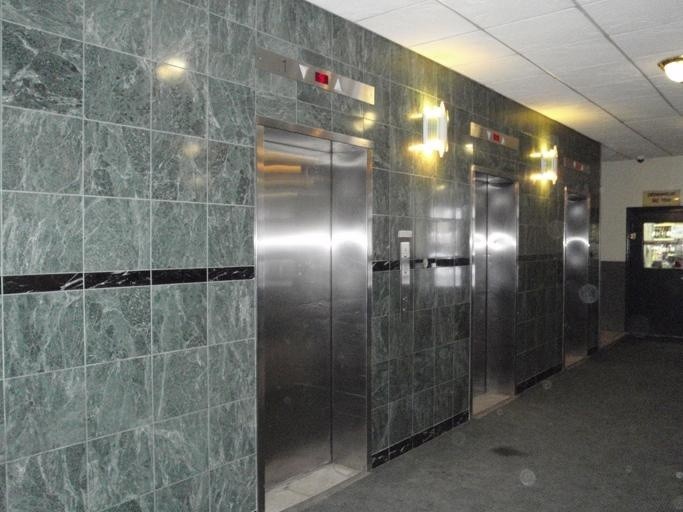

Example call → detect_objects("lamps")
404 100 451 158
531 143 560 186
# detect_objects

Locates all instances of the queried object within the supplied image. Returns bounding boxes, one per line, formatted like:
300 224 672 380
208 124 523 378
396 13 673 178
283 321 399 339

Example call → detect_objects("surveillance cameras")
636 155 645 163
